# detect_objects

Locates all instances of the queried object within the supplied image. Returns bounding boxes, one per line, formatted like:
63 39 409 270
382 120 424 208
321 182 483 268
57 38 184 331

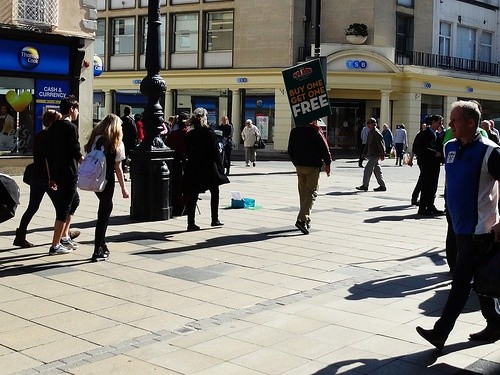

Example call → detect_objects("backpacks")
76 135 108 192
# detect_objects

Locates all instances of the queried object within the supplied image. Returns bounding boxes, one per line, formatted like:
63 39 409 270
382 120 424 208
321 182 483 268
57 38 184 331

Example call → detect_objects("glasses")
367 122 371 124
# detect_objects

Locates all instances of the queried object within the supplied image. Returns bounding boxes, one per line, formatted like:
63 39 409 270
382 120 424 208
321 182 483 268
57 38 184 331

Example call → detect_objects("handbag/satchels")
0 174 20 223
22 163 36 186
254 134 266 149
389 146 397 158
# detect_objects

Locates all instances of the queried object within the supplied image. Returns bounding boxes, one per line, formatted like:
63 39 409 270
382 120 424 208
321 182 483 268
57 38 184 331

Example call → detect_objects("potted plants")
344 23 368 45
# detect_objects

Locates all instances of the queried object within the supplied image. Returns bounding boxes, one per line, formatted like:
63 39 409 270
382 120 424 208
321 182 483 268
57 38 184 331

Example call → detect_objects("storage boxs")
232 198 254 208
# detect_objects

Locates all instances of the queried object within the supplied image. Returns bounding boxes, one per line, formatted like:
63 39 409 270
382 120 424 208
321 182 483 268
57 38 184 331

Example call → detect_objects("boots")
12 228 35 248
68 231 81 239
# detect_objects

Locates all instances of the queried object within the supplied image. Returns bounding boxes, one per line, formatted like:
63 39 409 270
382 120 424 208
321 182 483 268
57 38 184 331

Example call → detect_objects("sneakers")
49 243 74 255
60 236 78 250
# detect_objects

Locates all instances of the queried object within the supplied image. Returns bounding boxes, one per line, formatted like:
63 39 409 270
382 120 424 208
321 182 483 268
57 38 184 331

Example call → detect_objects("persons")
160 113 191 149
288 121 331 234
13 110 80 248
43 95 85 255
217 115 234 175
120 107 145 180
356 118 386 191
84 113 128 262
241 119 261 166
382 123 408 166
416 100 500 349
180 108 230 231
359 122 370 160
408 101 500 293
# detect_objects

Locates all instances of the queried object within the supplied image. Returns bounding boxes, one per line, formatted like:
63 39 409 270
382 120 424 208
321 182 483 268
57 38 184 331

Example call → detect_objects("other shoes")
426 205 444 215
91 244 111 262
211 220 224 226
469 327 500 340
374 186 386 191
246 162 256 167
295 219 311 234
416 326 448 350
356 185 368 191
187 223 200 230
412 199 420 206
418 209 434 218
